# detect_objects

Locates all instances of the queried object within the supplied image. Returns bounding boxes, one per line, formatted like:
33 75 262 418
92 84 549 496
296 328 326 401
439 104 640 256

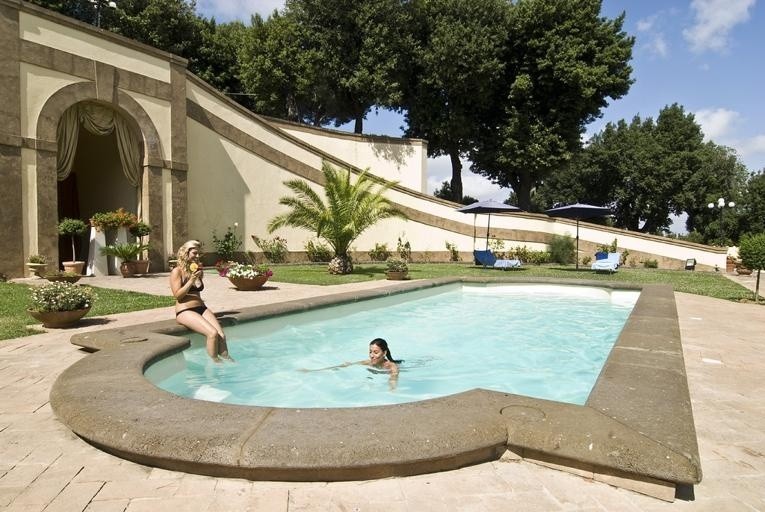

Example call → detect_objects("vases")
24 263 48 280
110 226 128 275
86 227 109 276
384 272 406 281
134 260 149 274
120 261 137 278
27 307 91 328
227 278 269 291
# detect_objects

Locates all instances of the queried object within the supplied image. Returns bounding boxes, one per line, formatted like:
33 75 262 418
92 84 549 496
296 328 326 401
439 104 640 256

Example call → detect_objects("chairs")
591 252 621 275
473 250 521 271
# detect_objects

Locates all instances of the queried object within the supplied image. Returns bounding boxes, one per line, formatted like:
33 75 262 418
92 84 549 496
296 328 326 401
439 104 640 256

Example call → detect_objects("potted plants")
55 217 88 275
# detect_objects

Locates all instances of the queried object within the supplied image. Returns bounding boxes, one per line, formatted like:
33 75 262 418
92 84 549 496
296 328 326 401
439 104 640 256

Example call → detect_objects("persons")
170 240 237 365
298 338 404 392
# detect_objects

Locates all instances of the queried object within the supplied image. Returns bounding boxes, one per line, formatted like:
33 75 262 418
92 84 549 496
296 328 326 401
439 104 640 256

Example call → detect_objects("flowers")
97 238 150 263
129 221 152 261
383 255 408 273
217 260 273 280
28 280 99 313
23 254 48 264
89 207 137 233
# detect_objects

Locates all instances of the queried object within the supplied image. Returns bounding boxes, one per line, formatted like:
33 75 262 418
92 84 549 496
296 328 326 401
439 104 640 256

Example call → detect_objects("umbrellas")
454 199 520 251
543 203 610 270
